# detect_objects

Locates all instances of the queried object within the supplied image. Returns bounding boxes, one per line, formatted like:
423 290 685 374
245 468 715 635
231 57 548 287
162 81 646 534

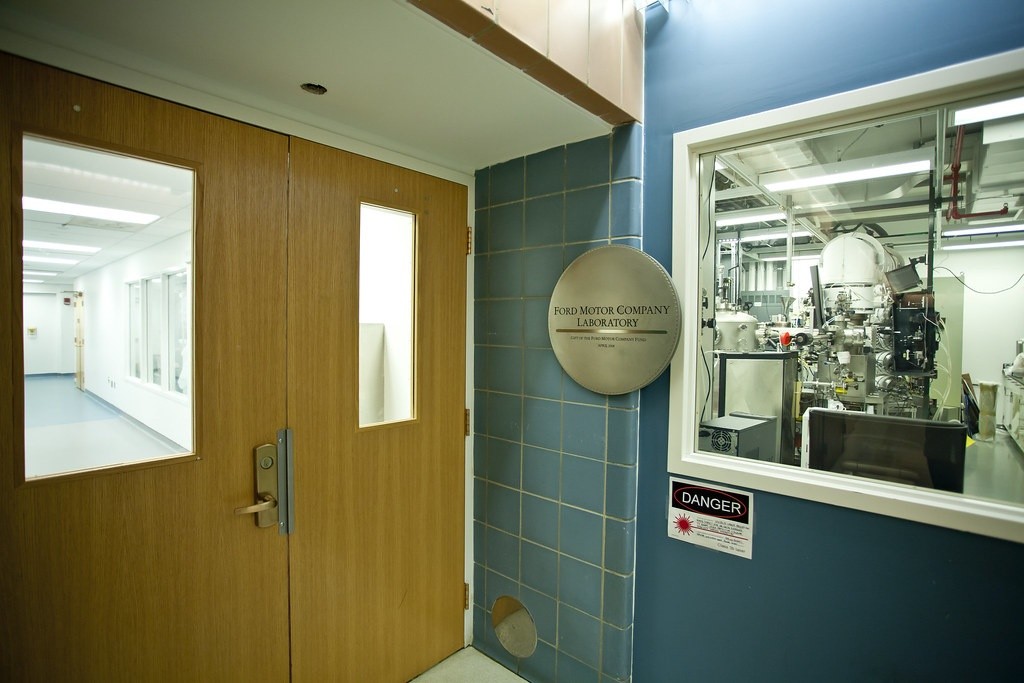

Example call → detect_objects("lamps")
942 220 1024 236
717 225 812 242
945 87 1024 128
714 205 785 226
759 249 822 261
758 145 936 193
940 234 1024 250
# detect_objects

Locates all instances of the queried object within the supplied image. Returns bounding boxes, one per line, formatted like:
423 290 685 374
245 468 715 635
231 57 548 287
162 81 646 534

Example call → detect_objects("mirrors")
667 47 1024 544
